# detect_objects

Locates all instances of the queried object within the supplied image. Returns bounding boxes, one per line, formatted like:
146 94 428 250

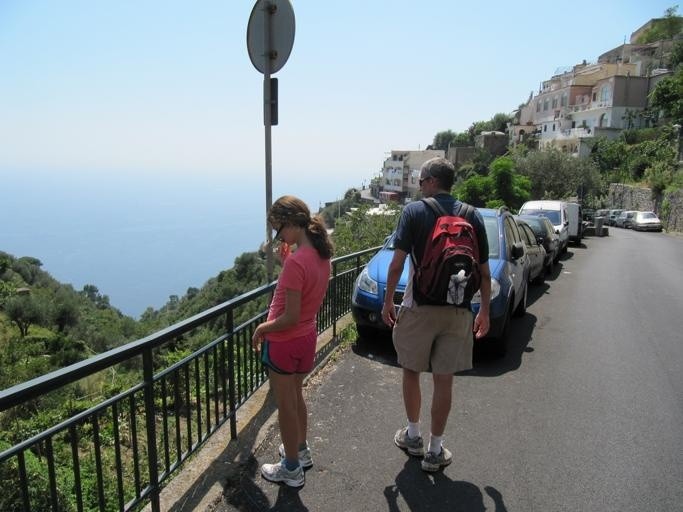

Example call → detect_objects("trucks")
566 203 582 247
518 200 569 258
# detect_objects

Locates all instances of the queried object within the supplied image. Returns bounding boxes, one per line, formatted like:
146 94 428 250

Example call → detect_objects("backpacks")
410 197 483 307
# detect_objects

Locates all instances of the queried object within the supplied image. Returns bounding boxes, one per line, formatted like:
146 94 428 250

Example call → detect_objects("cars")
350 206 531 355
582 208 594 222
614 211 640 229
514 215 560 276
603 209 625 226
513 217 548 286
630 211 662 232
591 209 612 226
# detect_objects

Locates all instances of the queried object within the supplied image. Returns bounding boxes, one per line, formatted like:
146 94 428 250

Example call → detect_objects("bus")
378 191 399 204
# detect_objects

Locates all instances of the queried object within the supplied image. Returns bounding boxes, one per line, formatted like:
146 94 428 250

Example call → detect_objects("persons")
251 194 334 489
380 157 491 473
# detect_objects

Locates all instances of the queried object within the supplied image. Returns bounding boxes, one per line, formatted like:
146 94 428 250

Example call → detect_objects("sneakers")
261 458 305 488
279 443 313 468
421 447 452 472
394 428 424 457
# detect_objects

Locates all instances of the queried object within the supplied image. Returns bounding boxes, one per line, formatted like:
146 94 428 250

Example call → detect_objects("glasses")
273 224 285 242
